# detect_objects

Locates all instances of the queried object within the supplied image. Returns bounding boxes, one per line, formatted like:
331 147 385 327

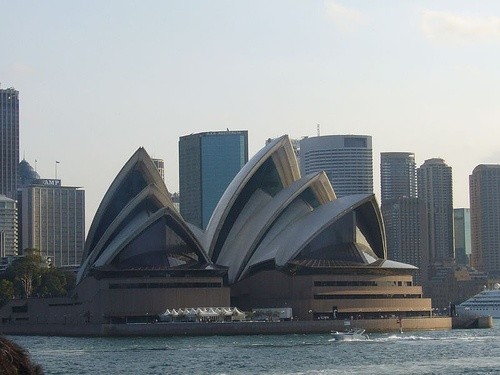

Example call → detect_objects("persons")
1 335 44 375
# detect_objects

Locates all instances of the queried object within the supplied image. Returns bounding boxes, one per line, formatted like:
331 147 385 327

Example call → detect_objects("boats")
455 288 500 318
330 324 366 341
